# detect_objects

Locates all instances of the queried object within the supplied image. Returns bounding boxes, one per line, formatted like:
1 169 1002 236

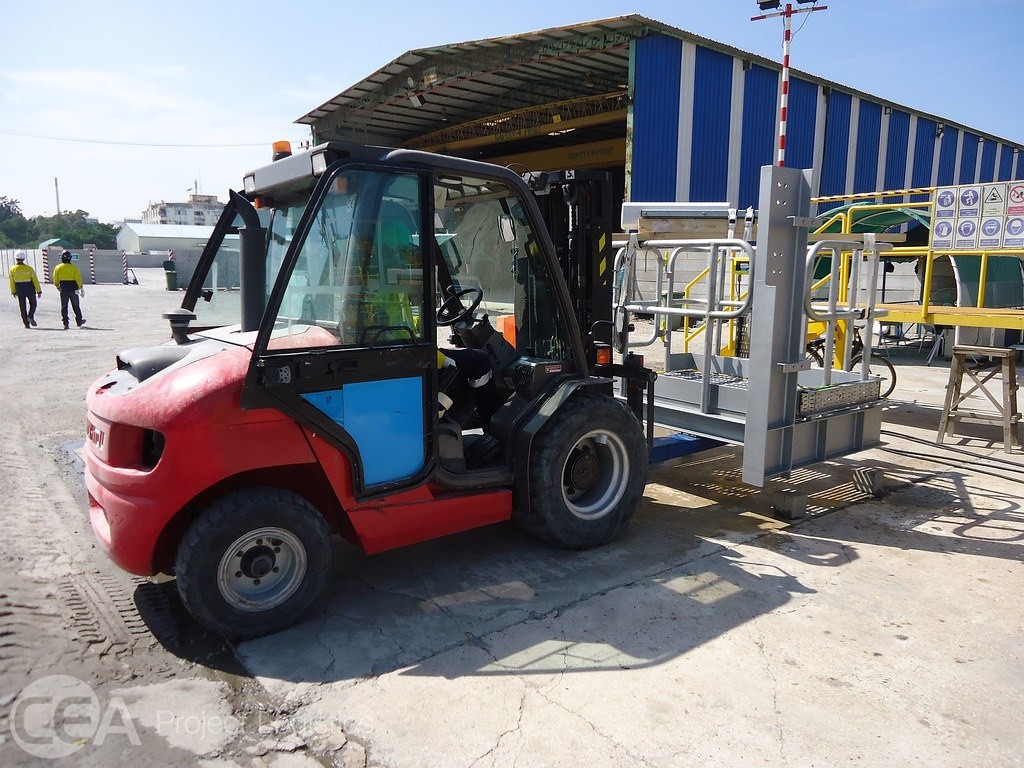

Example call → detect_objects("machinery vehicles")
81 139 733 645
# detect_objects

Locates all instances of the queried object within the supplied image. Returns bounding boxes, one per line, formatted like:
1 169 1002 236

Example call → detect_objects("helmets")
61 251 73 259
14 250 26 258
372 224 414 265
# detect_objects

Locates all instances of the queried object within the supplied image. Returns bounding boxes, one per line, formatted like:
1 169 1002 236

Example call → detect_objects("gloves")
80 288 85 297
444 357 456 369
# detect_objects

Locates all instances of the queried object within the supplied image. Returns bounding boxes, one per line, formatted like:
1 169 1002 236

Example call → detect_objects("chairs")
876 321 912 360
918 323 944 356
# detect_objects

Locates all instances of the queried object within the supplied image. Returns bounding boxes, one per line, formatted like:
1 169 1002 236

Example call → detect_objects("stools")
936 343 1023 452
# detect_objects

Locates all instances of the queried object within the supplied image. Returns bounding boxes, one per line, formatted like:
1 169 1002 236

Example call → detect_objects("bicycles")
803 310 896 401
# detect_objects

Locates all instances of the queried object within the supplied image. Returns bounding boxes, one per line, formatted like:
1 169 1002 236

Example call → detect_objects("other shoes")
27 315 37 327
77 319 86 327
64 325 69 330
25 322 31 328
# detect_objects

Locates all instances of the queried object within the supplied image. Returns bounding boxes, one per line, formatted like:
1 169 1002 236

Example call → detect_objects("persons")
53 251 87 329
8 251 42 329
341 200 493 434
